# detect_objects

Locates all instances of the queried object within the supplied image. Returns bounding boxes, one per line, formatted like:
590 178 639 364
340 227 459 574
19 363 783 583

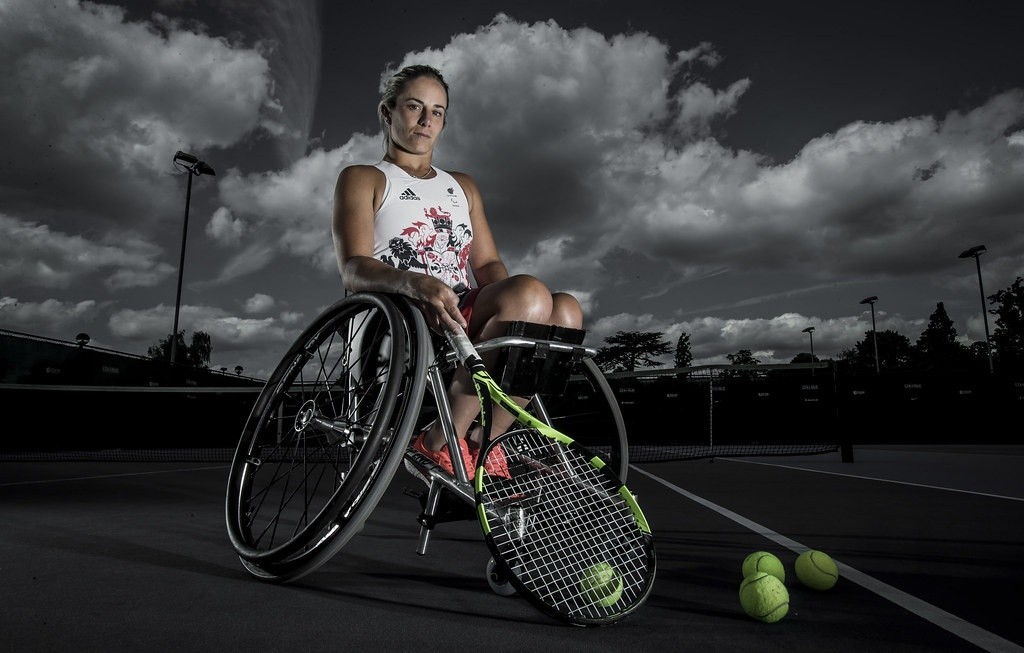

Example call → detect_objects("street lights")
802 327 815 377
170 151 217 367
859 296 881 376
957 245 995 375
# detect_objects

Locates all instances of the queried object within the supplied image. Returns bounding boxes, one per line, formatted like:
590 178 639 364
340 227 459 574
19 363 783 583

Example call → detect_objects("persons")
332 64 582 500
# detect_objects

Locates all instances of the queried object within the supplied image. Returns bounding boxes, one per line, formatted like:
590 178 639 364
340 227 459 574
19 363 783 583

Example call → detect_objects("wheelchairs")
224 292 630 584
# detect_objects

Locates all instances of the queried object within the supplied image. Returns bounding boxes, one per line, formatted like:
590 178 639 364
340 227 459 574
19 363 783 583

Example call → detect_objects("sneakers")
468 442 524 501
411 430 475 482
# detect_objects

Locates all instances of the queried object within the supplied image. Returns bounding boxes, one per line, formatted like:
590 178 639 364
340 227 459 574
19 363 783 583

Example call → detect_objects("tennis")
580 562 624 608
740 550 787 586
794 547 842 594
737 570 791 625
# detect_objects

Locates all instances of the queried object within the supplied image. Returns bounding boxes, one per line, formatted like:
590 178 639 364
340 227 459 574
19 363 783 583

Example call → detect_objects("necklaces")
413 168 432 178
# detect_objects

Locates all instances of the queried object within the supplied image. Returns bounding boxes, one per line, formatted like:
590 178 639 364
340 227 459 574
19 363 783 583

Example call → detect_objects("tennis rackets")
439 314 658 628
